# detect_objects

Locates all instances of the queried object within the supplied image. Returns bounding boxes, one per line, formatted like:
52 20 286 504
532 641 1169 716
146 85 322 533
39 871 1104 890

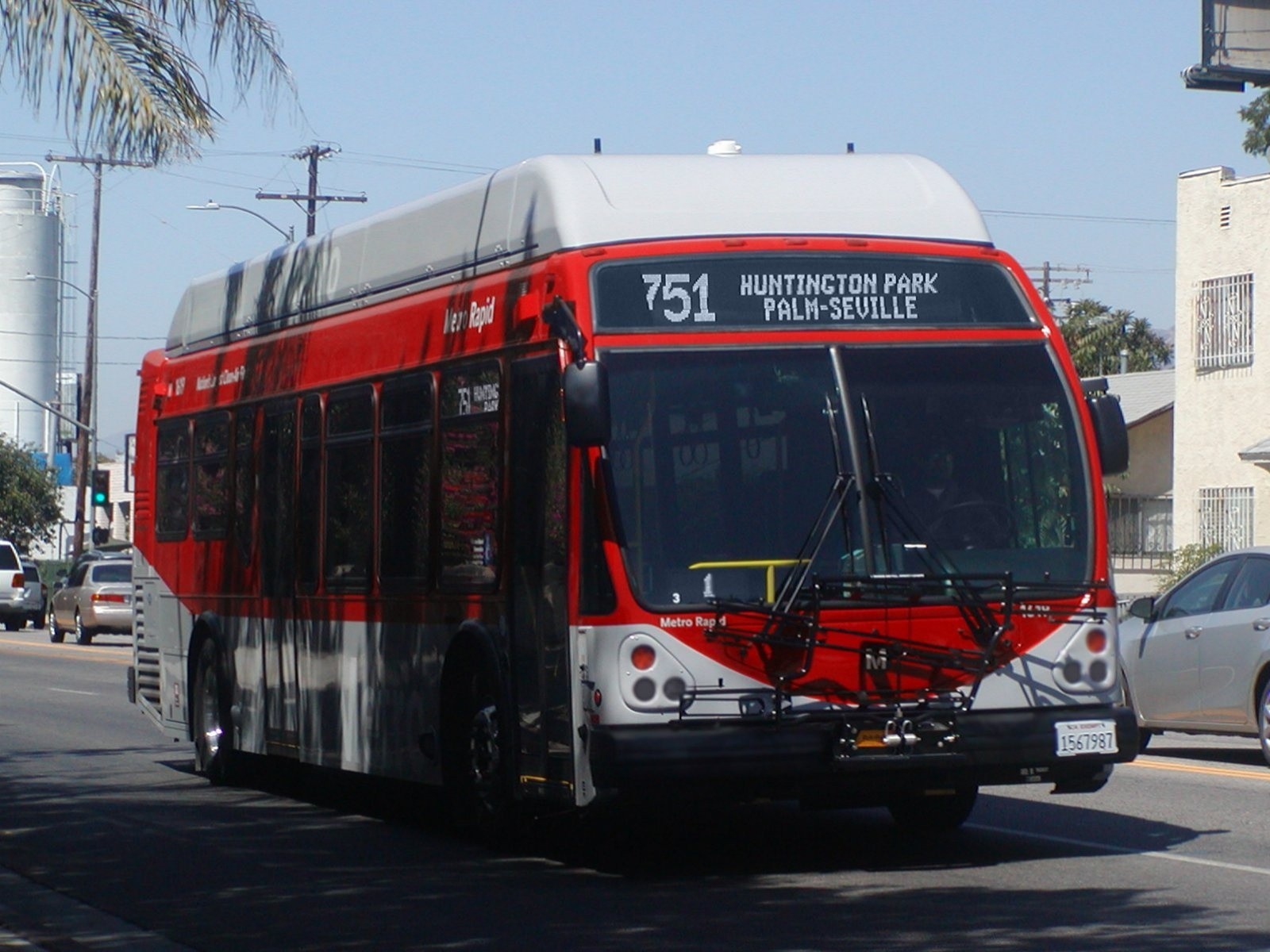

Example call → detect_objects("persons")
895 440 1013 551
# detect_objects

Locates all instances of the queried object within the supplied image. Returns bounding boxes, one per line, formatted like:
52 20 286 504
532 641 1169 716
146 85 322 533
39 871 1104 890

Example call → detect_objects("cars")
1118 543 1270 764
0 539 27 632
59 550 132 589
47 559 133 646
20 562 47 629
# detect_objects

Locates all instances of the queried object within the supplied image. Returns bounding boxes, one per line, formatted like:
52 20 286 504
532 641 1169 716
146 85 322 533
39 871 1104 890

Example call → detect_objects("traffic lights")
91 469 111 506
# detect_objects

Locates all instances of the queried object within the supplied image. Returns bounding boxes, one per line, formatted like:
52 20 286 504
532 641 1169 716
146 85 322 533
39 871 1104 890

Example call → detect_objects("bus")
125 136 1131 830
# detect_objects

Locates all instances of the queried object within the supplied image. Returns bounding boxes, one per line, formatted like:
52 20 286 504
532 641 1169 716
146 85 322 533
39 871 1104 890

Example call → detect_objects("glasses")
925 452 953 463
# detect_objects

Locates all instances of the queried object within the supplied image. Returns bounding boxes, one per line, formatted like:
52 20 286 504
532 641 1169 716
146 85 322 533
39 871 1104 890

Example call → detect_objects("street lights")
0 271 101 557
